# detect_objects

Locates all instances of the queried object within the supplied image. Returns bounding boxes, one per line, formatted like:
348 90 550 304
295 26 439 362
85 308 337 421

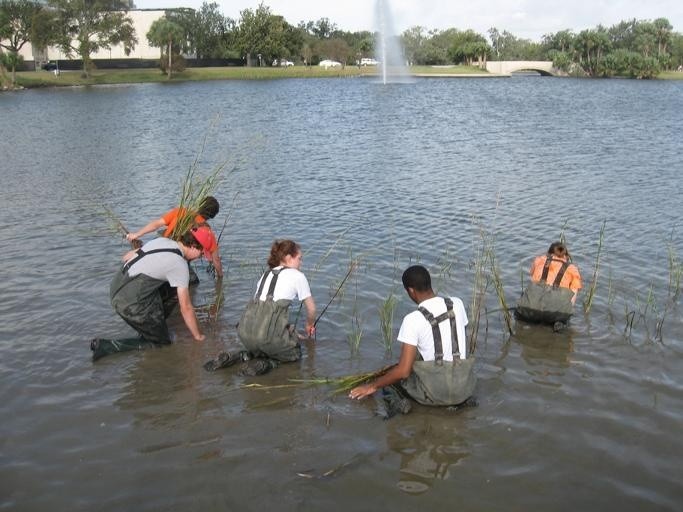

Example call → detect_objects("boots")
457 397 481 414
91 336 140 363
553 320 567 335
202 347 247 371
236 357 276 378
379 385 411 422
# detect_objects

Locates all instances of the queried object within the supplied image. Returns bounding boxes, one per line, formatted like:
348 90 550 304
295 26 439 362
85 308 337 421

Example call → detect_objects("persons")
203 239 316 377
126 196 224 283
514 241 583 333
348 266 479 421
89 225 215 365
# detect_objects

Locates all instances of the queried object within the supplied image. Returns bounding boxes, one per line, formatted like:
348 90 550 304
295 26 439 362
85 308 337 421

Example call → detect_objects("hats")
191 226 218 260
